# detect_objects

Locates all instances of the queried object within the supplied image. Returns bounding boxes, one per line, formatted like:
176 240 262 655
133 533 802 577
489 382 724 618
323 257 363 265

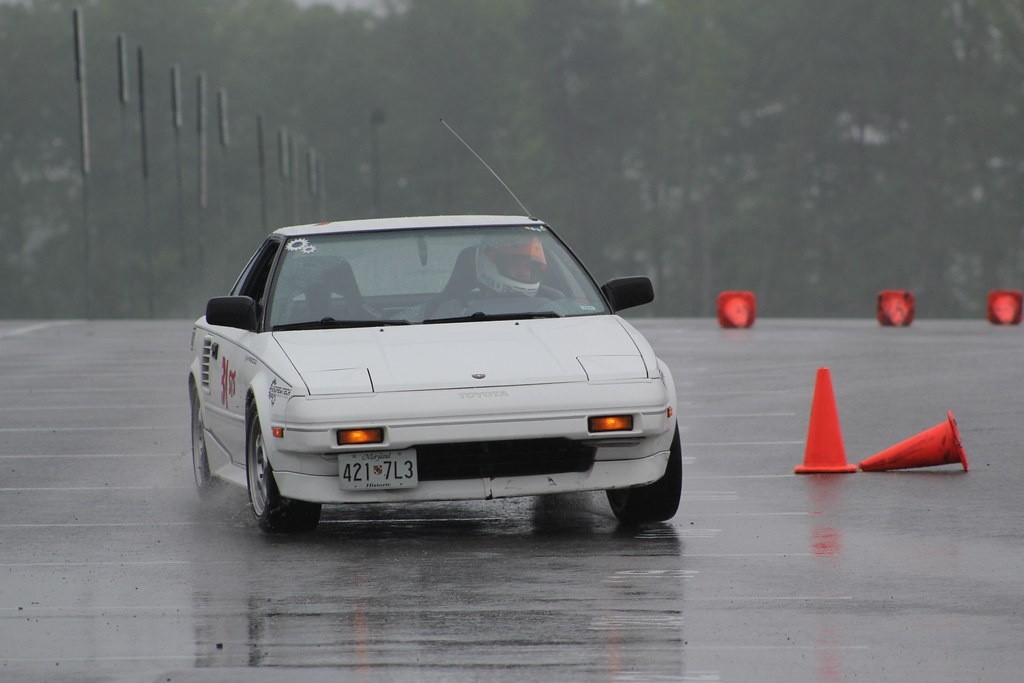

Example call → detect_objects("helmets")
475 226 546 299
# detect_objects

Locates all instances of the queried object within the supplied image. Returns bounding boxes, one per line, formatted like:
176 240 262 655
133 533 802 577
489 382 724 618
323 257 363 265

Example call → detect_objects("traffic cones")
985 289 1024 327
717 289 757 329
876 289 916 327
792 366 860 473
856 408 972 474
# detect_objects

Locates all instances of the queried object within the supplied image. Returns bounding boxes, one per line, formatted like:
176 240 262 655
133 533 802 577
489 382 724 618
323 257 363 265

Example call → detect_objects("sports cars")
186 210 684 536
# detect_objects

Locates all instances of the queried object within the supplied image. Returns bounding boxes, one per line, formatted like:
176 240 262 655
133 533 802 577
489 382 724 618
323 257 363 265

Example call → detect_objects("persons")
445 230 570 319
